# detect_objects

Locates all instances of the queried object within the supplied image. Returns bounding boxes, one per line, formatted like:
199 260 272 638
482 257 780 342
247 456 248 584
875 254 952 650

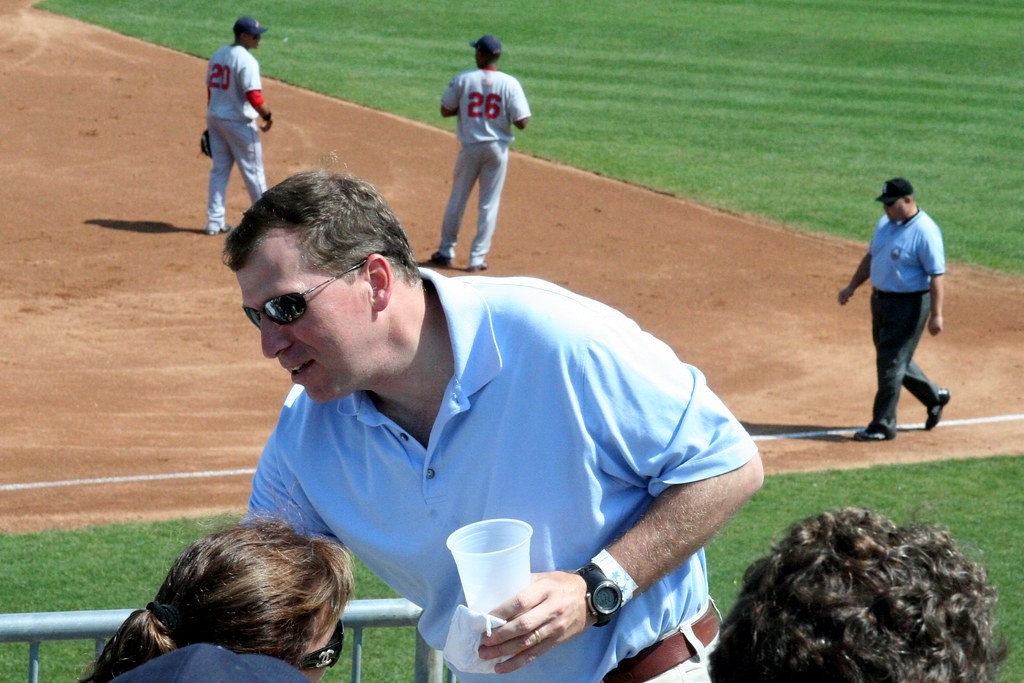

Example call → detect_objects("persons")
203 15 276 239
428 32 534 270
70 518 353 681
707 506 1012 683
834 177 951 443
219 162 766 681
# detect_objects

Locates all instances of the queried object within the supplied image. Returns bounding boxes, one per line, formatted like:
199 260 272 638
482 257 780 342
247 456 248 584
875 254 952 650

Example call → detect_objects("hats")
875 178 913 204
469 35 502 59
233 16 268 34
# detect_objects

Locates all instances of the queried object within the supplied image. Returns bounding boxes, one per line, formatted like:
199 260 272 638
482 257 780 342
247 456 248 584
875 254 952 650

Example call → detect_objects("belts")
602 599 719 683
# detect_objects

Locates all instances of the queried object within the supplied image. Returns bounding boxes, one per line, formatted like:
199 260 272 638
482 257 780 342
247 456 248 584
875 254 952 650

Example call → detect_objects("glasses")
242 257 368 331
300 618 344 671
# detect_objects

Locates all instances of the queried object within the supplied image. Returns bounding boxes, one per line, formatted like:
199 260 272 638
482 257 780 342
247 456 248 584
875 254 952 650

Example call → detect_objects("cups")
445 518 533 616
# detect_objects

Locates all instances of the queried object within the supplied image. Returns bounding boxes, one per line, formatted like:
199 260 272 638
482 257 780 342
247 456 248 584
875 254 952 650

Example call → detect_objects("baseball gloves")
201 129 212 158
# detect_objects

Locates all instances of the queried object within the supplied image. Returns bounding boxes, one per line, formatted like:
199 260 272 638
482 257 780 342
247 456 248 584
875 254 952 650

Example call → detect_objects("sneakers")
469 263 487 271
208 223 230 236
431 252 450 265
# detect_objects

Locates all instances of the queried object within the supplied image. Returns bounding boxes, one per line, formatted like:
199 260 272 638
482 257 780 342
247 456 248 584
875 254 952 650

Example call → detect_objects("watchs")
579 560 625 629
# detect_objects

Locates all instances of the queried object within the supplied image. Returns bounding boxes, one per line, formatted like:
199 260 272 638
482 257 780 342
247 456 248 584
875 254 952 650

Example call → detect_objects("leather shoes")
854 428 897 440
925 387 950 430
